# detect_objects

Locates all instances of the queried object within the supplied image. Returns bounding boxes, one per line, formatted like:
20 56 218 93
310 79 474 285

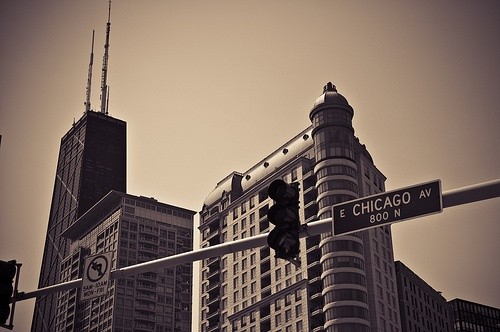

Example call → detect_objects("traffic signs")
331 179 443 238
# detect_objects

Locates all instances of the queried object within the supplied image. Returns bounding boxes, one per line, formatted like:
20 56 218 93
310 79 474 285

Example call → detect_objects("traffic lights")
266 178 301 260
0 259 17 326
